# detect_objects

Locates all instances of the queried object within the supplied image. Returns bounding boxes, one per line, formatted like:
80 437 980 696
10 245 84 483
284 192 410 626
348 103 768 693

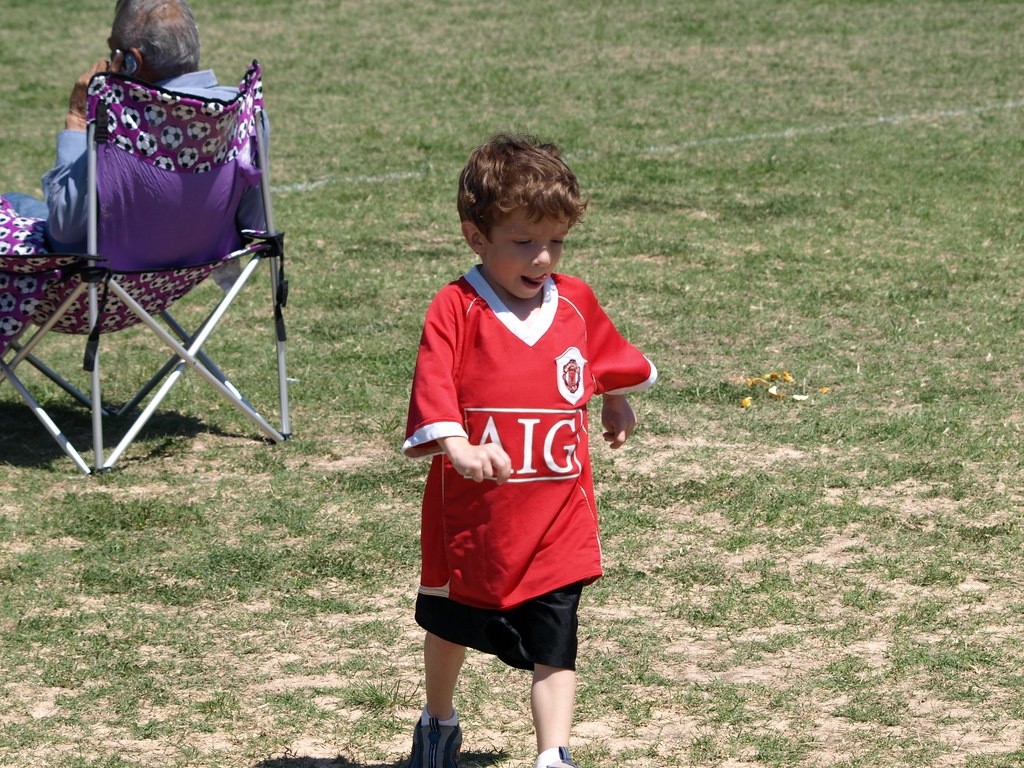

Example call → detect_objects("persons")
401 128 658 768
0 0 270 352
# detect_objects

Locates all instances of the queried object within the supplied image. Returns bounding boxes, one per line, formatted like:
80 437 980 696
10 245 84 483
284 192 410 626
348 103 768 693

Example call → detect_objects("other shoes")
409 718 463 768
537 747 581 768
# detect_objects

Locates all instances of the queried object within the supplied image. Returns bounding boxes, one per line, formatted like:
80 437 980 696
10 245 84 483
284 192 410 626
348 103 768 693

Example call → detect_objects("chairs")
0 60 291 475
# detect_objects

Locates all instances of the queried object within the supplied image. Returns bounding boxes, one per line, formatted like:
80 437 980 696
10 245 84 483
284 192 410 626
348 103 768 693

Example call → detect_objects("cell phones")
120 55 136 77
